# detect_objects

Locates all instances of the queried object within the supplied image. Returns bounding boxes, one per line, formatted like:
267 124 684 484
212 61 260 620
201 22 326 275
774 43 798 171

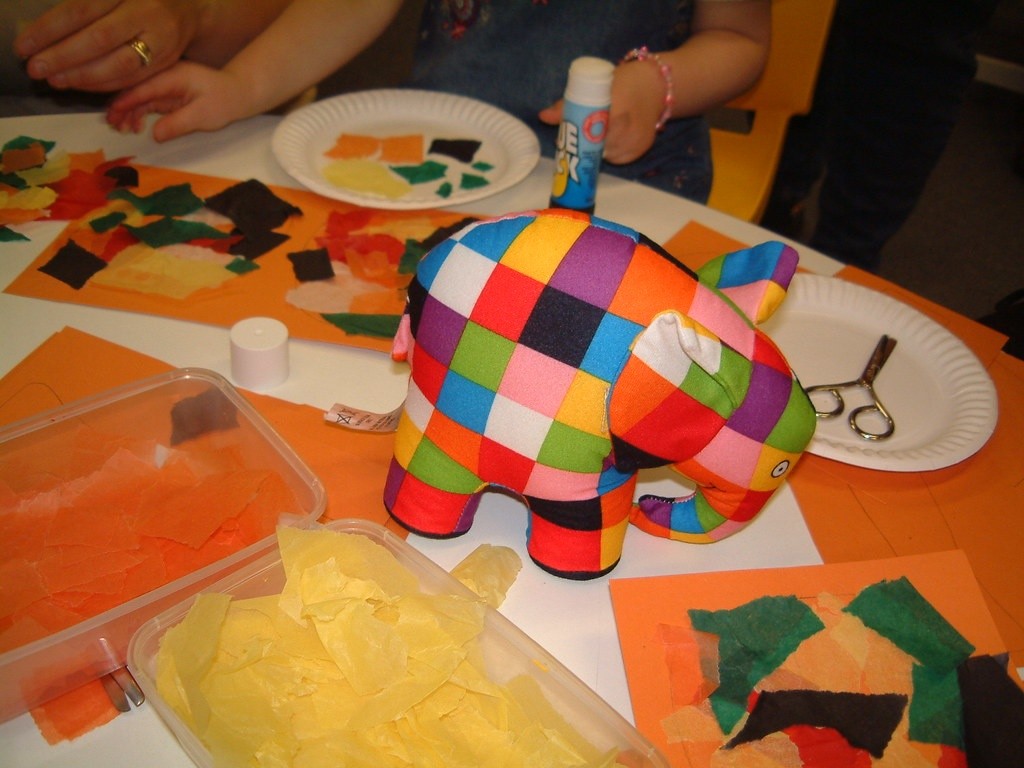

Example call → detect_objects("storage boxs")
127 520 670 768
0 366 330 725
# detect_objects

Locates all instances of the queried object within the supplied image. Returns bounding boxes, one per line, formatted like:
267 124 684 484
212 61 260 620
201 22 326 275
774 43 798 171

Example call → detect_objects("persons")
749 0 986 269
13 2 769 205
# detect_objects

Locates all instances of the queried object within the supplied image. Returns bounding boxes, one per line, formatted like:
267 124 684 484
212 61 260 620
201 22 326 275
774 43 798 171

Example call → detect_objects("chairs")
701 0 838 225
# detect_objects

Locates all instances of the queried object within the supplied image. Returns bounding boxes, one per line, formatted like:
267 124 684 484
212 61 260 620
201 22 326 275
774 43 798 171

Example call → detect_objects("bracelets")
622 42 676 135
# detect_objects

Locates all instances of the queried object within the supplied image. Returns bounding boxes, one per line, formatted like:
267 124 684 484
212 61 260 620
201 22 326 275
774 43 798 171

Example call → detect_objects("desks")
0 111 1024 768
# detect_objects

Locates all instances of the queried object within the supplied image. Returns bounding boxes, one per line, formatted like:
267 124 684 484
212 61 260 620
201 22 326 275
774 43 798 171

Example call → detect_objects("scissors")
805 333 899 441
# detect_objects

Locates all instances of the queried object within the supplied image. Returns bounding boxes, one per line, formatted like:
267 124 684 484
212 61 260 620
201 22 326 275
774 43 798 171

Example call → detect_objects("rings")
127 38 154 70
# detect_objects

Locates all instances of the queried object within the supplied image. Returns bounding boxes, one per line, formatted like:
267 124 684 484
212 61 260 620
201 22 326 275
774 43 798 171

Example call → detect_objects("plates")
269 89 543 210
748 273 998 472
